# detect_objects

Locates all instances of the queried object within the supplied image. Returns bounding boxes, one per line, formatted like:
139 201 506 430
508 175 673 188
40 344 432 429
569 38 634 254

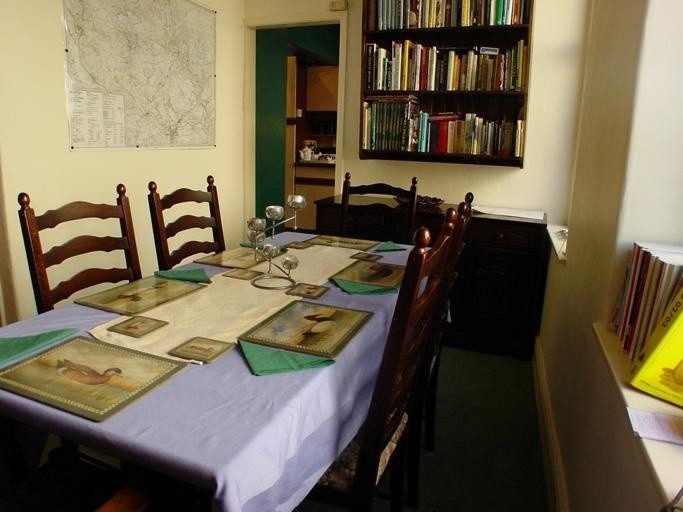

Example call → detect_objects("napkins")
0 328 79 365
154 268 213 284
237 339 335 377
241 242 288 251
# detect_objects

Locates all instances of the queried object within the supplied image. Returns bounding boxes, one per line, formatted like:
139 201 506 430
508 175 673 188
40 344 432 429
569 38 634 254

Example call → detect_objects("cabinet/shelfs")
359 0 535 169
310 190 554 358
294 165 334 232
305 64 338 111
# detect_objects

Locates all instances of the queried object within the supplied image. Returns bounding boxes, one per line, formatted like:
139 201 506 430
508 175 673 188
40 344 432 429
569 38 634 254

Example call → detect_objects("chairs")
308 172 473 511
147 175 226 271
18 184 143 315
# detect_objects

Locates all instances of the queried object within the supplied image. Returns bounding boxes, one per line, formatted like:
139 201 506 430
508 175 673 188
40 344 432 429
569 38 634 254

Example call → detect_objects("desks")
0 232 432 512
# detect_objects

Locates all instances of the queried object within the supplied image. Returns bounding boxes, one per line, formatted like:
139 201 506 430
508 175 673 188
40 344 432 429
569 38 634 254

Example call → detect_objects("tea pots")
299 145 314 160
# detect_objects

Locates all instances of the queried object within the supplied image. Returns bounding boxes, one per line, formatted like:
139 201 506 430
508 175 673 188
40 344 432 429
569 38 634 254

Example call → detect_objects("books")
606 240 683 408
362 1 529 158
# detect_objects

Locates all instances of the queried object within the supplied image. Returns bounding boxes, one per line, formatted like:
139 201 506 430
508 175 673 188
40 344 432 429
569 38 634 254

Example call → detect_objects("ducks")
362 265 395 281
303 313 337 337
55 358 124 386
116 280 168 302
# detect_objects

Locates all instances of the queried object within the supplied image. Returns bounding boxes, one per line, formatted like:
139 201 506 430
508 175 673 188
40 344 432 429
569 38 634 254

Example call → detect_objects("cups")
296 108 302 117
